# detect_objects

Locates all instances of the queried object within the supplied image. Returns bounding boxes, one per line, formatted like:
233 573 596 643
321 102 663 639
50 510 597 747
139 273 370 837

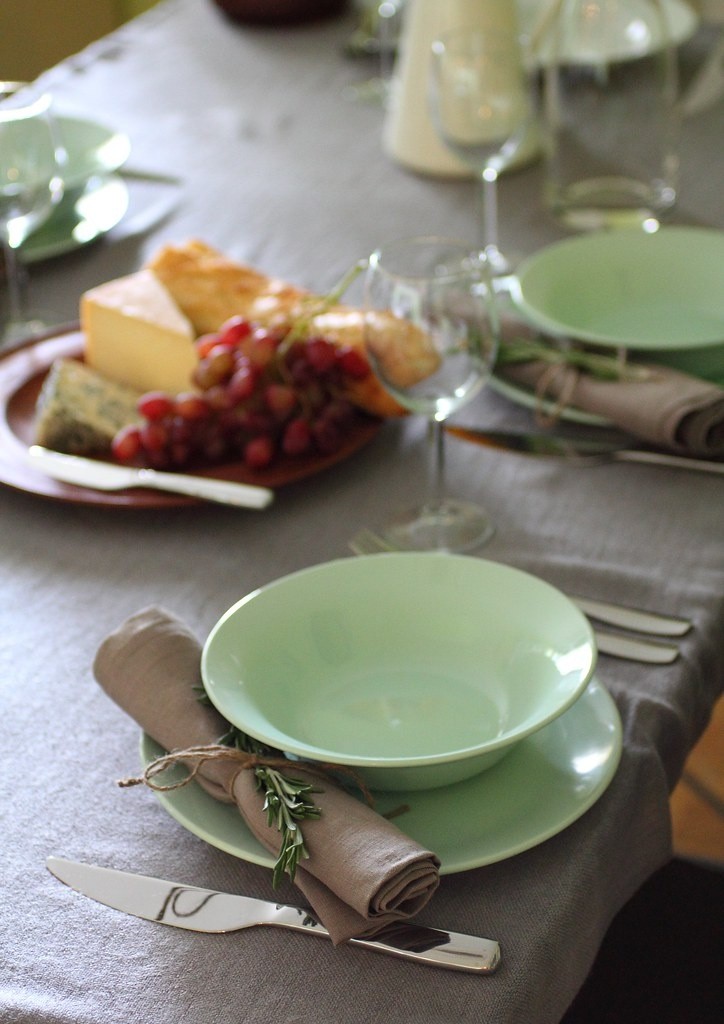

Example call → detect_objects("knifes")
44 857 502 975
28 444 276 510
444 425 724 473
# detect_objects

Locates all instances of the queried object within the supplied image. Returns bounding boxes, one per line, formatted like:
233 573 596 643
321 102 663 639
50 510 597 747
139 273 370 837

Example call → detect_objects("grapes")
107 315 374 465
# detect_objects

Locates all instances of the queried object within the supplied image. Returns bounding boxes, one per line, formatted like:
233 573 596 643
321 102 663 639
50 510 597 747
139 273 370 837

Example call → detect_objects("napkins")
422 298 724 459
93 602 444 949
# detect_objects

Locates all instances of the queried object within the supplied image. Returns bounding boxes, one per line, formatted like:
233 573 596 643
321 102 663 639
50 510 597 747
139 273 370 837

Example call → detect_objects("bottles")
382 1 545 180
536 1 684 231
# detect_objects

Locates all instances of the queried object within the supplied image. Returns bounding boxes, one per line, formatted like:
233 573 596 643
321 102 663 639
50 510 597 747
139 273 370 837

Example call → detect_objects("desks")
0 0 724 1024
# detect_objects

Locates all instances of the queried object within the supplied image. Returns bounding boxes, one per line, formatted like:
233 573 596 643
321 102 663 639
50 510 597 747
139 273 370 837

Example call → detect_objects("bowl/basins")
0 113 126 223
199 550 598 792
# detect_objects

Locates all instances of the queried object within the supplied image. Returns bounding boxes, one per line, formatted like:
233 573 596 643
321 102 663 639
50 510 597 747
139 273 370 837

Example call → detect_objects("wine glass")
424 26 536 293
360 235 501 551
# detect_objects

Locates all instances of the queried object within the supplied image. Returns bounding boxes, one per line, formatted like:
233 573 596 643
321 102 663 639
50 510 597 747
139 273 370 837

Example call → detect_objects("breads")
149 239 440 383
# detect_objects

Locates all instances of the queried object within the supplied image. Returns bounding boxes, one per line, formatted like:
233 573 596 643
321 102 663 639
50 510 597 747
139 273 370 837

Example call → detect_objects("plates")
470 297 724 425
518 0 696 67
0 318 381 505
507 224 724 352
0 173 129 259
139 677 623 873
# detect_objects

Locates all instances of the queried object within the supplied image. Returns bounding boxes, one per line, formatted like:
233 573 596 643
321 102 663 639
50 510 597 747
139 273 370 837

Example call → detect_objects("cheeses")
78 270 204 400
33 360 154 454
